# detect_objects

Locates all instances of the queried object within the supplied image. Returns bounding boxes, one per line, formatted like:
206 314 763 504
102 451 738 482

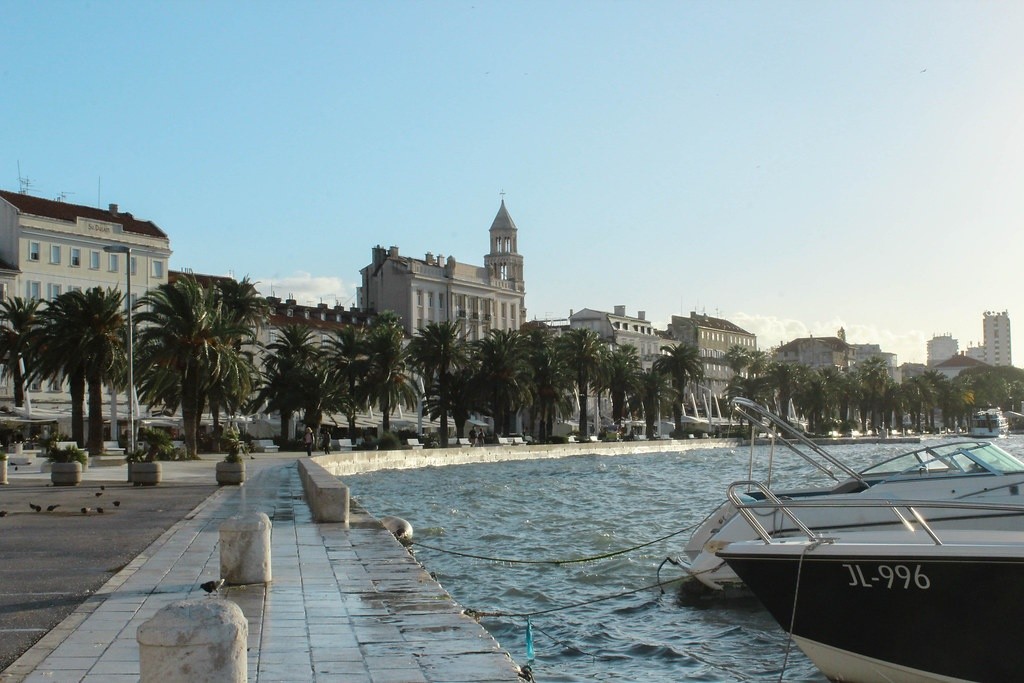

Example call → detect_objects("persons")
320 425 325 434
630 427 634 441
318 431 331 454
304 427 314 456
469 426 485 447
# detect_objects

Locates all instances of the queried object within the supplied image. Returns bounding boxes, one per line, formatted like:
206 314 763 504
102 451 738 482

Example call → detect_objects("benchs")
103 441 126 455
567 437 579 444
515 437 528 445
337 439 358 451
634 433 712 441
498 438 512 446
55 442 85 451
458 438 472 447
758 429 963 438
22 450 42 459
448 438 458 444
589 436 602 443
239 440 280 453
407 439 425 449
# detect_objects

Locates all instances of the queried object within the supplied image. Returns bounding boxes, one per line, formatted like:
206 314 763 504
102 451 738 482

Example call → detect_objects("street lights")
103 244 135 463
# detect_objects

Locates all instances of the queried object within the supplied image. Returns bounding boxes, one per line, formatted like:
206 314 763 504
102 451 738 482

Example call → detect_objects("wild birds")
0 484 121 517
198 579 225 596
10 462 32 471
251 456 255 459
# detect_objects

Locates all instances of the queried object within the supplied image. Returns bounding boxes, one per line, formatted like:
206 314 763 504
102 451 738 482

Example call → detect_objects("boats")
970 407 1009 437
714 480 1024 683
680 390 808 437
666 397 1024 595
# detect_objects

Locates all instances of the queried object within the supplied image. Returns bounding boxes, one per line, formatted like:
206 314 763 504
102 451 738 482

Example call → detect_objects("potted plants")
125 426 175 485
48 445 88 486
4 420 49 457
0 450 10 485
216 426 246 486
